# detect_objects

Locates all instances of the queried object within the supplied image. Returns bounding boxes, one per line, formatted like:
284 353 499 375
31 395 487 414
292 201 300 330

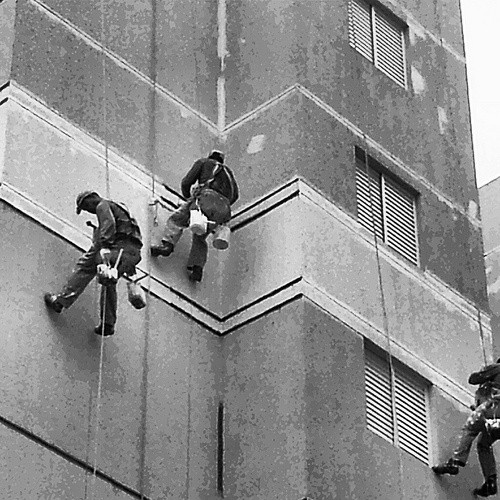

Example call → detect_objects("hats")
208 149 226 164
76 190 95 215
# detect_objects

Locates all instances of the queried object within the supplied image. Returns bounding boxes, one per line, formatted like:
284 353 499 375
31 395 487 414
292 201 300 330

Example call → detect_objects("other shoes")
151 242 174 257
473 479 497 498
94 322 115 336
188 270 202 282
431 458 460 475
44 293 64 313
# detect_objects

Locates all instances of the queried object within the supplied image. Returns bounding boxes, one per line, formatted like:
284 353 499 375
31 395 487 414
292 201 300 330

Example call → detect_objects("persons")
149 149 239 282
432 356 500 497
44 190 143 336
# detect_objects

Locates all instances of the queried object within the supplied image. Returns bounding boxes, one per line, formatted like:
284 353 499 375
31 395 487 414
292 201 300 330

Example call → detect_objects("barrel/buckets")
126 282 147 309
212 223 231 250
189 209 208 236
